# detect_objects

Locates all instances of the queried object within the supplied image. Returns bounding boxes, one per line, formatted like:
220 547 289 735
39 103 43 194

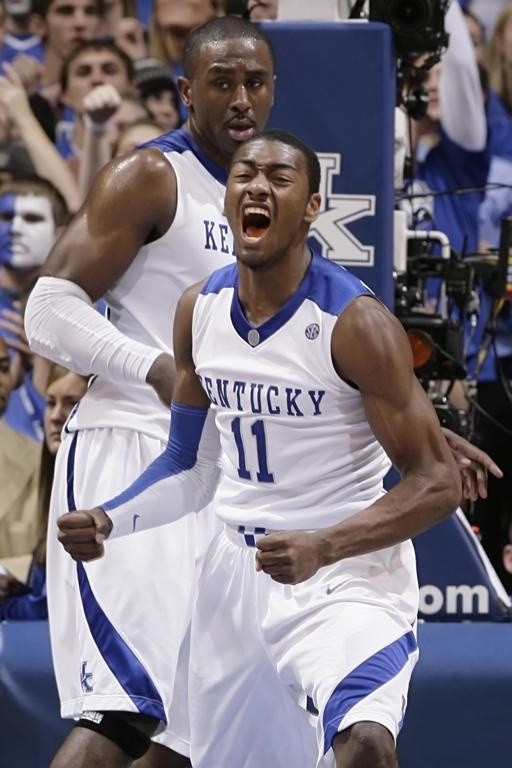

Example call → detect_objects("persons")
57 129 463 768
23 15 503 768
0 1 511 623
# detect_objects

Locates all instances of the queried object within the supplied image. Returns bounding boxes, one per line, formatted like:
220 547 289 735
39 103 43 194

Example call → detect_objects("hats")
132 58 174 85
